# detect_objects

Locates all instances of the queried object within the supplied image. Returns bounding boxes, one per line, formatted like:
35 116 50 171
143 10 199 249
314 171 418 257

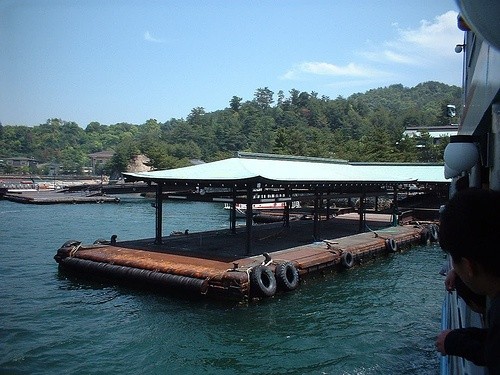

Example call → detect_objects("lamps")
454 44 465 52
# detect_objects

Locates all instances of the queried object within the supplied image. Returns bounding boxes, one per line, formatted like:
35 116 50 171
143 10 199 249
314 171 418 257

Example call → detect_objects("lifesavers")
276 261 299 290
341 252 354 268
93 239 109 244
388 239 398 252
61 240 81 247
250 265 278 296
421 229 432 244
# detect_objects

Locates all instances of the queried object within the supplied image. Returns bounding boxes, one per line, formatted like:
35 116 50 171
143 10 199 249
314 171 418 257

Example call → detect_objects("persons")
389 203 397 227
435 184 499 372
